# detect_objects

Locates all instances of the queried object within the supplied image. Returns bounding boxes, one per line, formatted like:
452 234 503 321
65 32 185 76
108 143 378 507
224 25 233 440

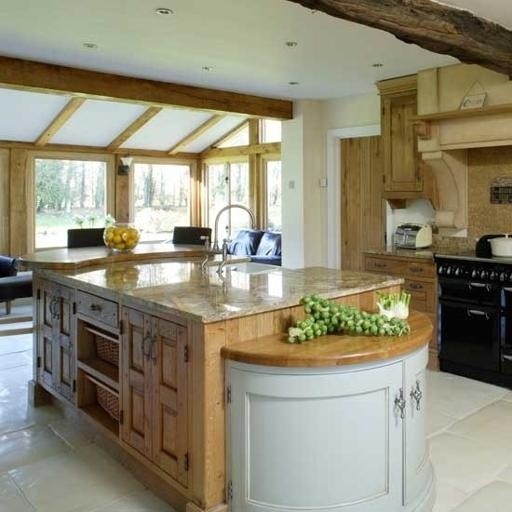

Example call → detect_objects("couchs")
228 228 281 267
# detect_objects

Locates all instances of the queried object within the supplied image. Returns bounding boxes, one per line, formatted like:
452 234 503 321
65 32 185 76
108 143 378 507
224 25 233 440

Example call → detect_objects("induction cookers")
433 253 512 283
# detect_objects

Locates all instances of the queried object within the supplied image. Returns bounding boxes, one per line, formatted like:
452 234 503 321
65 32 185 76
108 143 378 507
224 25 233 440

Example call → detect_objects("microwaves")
393 222 433 251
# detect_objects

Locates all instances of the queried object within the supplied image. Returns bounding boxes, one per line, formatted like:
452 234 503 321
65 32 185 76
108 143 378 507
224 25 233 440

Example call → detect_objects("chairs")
162 226 212 248
67 227 106 249
0 255 32 315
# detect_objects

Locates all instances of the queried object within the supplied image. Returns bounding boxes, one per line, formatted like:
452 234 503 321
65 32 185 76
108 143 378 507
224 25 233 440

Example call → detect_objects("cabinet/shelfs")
223 343 437 511
379 89 424 200
440 276 501 387
364 256 438 351
75 289 118 443
37 278 74 404
121 305 188 488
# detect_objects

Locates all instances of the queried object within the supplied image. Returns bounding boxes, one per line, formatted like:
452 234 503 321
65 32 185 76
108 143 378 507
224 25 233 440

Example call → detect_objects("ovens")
437 277 512 390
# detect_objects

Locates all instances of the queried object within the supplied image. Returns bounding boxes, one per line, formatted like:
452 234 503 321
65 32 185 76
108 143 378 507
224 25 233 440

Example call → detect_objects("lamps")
118 156 134 175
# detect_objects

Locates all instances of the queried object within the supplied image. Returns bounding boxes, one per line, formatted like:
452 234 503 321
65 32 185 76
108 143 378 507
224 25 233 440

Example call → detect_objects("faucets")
206 200 258 258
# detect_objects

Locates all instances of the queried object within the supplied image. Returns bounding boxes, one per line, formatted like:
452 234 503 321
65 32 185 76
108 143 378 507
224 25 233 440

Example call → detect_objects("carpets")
0 296 33 355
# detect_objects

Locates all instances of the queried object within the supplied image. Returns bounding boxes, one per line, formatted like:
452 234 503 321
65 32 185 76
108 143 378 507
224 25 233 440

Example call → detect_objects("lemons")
105 227 138 250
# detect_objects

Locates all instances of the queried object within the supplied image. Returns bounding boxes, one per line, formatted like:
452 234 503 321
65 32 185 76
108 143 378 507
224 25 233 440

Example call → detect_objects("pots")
487 234 512 257
